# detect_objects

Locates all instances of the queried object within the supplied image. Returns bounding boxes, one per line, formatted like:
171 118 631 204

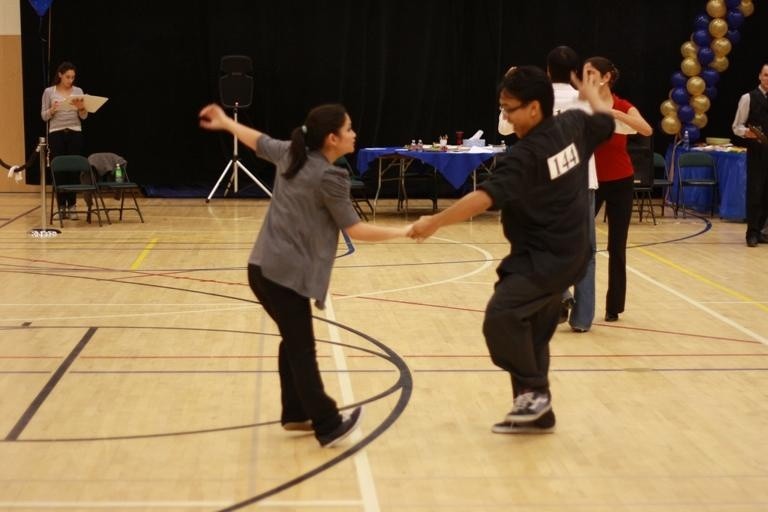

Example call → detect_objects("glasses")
499 106 522 115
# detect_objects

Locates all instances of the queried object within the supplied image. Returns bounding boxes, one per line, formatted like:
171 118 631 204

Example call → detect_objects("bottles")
500 140 505 151
683 131 689 151
114 164 124 182
417 140 423 152
410 140 416 152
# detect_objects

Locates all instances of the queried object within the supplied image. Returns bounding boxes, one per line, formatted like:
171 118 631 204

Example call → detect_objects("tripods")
204 108 274 203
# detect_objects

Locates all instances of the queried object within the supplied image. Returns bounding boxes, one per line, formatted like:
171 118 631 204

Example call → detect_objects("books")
53 92 109 114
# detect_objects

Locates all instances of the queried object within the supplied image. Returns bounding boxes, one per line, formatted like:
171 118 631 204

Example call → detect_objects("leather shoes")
747 233 768 247
557 298 576 324
605 311 619 321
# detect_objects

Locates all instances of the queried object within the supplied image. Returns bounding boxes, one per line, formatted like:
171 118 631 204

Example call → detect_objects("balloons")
656 0 757 146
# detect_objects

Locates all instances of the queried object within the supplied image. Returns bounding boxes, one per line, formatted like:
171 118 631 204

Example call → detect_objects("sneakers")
53 205 66 219
315 406 362 447
491 390 556 433
68 203 79 220
281 410 315 431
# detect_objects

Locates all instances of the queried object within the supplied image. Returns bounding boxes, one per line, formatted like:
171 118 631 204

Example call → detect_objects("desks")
661 140 751 220
352 145 516 223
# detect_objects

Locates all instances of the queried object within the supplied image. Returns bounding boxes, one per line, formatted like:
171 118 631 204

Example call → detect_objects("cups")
488 144 492 151
439 140 448 152
456 128 464 145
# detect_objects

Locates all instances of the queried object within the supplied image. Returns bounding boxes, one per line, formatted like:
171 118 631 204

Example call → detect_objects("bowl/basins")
706 138 732 145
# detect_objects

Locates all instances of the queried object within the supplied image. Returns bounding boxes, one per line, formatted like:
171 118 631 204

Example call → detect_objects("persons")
39 61 88 221
495 41 639 336
728 62 767 249
405 62 617 436
197 98 415 451
578 55 653 326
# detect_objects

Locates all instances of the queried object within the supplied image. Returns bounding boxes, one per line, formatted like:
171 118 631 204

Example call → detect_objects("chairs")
334 156 376 222
602 146 661 227
635 151 678 219
674 153 729 223
84 151 148 225
47 154 107 228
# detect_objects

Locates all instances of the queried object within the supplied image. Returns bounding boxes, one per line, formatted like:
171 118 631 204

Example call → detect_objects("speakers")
220 56 255 108
629 134 653 188
